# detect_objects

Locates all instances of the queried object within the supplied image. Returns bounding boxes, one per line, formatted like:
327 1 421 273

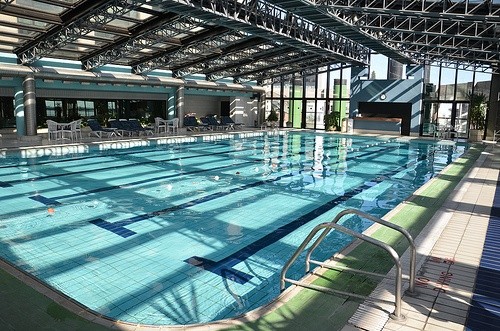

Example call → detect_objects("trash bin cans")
342 118 347 134
347 118 354 133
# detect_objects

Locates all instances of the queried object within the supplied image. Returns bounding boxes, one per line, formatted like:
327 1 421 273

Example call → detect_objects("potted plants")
324 111 340 131
267 109 278 128
466 90 489 143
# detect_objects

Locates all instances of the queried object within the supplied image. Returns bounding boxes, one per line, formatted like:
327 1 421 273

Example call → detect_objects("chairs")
46 119 82 142
155 117 179 135
86 118 154 139
184 113 245 133
